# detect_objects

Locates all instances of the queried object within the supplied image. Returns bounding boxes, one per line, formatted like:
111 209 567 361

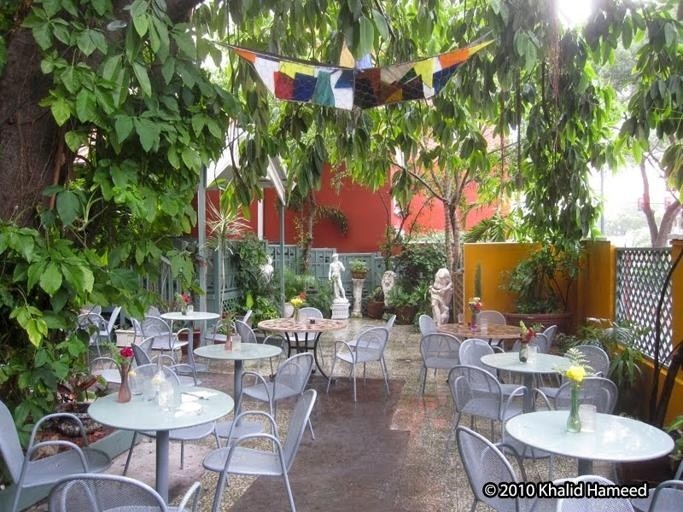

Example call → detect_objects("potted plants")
504 244 582 335
366 287 385 319
349 261 371 278
392 292 421 322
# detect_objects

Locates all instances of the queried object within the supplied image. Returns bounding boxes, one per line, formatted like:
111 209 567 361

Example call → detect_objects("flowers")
290 293 308 308
175 292 191 304
469 297 482 312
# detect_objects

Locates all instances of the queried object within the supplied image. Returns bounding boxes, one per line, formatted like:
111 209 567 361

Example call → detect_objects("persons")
430 268 452 326
328 253 346 299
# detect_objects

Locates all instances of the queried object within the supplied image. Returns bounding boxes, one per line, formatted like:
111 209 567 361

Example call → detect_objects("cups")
142 376 156 401
480 318 488 330
232 336 241 352
528 344 538 362
458 313 465 327
159 382 173 412
188 305 193 315
130 374 141 394
